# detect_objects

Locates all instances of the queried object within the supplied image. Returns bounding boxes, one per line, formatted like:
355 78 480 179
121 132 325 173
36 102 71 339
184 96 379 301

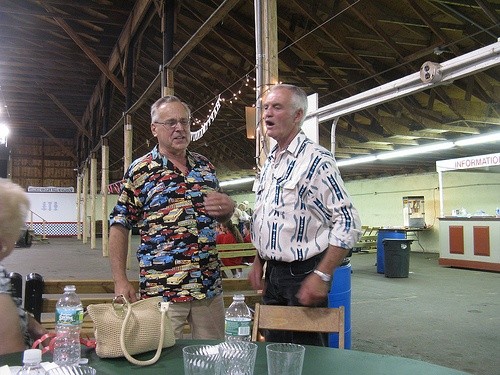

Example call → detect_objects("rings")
218 205 222 210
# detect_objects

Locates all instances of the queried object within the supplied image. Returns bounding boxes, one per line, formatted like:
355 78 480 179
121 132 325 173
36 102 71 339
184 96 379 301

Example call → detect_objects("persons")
214 201 255 278
0 178 53 355
109 95 237 339
248 84 361 346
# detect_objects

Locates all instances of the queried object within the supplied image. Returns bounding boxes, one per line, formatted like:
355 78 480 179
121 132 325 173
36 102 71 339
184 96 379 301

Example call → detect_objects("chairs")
251 303 344 349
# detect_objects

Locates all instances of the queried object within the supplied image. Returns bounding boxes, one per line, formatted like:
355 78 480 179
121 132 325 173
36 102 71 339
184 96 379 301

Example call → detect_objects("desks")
0 339 467 375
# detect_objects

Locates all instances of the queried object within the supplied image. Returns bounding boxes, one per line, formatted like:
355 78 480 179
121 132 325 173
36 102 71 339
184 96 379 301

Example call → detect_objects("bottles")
53 285 84 366
224 293 251 342
17 349 50 375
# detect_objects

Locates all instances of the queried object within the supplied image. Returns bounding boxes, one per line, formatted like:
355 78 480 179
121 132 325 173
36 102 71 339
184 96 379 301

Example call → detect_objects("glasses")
154 119 190 127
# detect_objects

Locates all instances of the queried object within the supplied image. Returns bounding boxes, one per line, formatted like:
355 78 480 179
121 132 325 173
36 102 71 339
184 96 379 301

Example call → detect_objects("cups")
182 344 218 375
217 340 258 375
266 343 306 375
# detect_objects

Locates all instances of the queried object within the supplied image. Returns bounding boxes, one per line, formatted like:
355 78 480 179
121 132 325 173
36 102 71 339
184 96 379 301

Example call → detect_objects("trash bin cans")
382 239 414 278
327 258 351 350
377 228 407 274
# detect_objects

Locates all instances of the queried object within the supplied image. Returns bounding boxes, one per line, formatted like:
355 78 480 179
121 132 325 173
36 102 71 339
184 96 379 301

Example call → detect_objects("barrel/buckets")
326 258 351 350
382 238 412 277
377 229 407 274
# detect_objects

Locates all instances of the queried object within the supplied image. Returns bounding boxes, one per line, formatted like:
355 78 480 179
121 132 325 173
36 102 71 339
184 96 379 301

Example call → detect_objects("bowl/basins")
45 365 96 375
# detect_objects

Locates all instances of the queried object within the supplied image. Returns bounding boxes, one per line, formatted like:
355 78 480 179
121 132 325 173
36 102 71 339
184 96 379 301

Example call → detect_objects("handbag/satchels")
87 294 175 366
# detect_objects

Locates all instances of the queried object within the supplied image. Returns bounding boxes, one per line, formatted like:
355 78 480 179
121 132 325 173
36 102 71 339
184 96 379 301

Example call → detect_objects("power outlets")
435 187 439 190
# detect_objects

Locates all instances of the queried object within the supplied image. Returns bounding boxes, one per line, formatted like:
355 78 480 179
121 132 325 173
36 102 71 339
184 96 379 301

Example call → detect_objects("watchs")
313 269 332 282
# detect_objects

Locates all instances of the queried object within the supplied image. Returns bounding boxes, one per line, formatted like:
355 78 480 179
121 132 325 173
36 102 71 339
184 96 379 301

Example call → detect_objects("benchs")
41 279 265 333
358 227 379 252
